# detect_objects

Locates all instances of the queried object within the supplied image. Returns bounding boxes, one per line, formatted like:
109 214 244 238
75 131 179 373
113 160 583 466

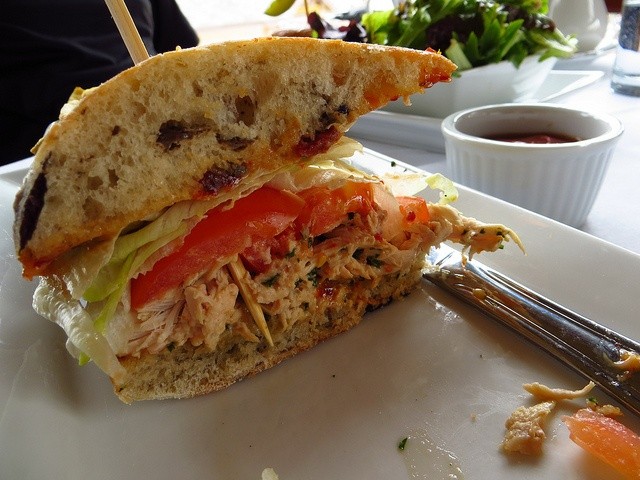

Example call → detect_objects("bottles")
549 1 607 52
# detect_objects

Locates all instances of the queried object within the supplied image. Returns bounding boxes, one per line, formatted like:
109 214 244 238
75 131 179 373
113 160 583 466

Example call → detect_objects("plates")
0 148 640 480
344 69 605 156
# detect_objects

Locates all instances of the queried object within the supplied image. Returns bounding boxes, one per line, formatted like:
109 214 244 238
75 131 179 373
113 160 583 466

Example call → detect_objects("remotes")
610 0 640 97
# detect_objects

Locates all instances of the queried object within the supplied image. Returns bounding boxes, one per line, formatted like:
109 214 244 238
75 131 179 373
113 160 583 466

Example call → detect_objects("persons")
1 0 199 166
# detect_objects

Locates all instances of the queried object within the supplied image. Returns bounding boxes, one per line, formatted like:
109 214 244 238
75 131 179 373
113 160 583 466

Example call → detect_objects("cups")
441 100 623 231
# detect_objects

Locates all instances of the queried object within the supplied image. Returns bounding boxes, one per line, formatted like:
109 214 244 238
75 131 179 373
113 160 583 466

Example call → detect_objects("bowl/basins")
383 50 556 115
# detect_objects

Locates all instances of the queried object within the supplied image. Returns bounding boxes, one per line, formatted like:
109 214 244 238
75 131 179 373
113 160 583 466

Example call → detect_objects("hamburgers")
11 37 531 402
